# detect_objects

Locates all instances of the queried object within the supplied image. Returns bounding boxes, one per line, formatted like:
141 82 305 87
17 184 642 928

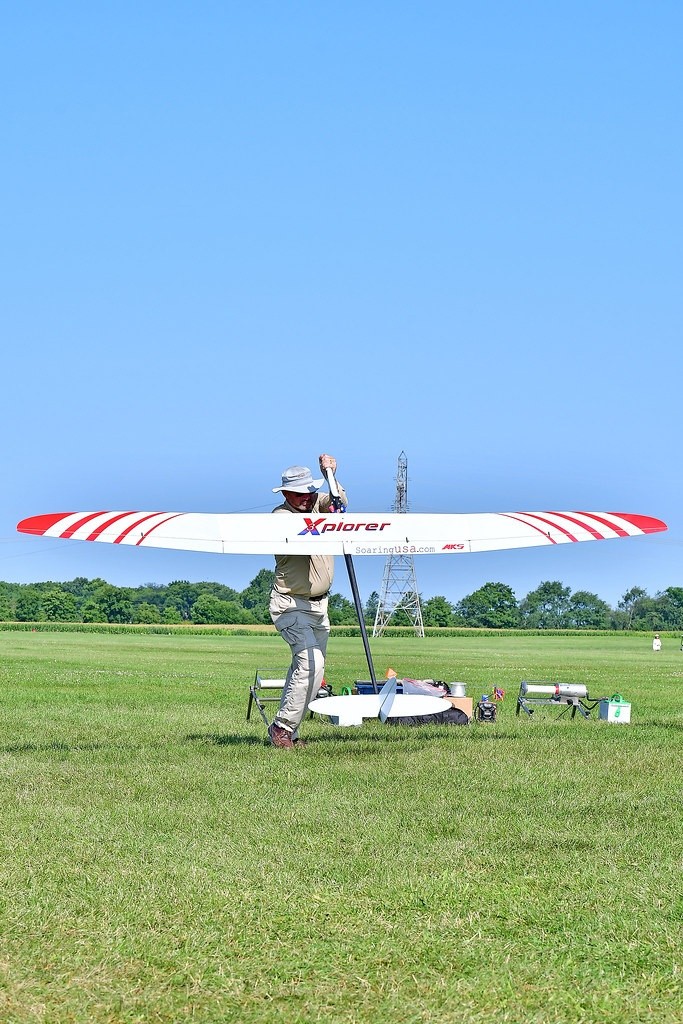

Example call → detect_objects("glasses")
296 492 315 497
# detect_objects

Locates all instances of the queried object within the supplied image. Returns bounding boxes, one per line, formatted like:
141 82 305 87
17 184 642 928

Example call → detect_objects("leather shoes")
294 739 308 747
268 723 295 750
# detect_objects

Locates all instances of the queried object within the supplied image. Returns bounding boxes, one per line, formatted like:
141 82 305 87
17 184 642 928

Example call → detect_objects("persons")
268 454 348 751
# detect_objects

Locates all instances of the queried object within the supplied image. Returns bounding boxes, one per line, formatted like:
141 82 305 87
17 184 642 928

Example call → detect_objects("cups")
450 681 466 697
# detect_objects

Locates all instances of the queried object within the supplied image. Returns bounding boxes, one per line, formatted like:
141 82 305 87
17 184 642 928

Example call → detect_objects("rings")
330 460 332 464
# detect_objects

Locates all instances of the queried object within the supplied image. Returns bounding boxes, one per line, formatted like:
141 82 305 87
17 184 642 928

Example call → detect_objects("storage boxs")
444 696 474 722
355 685 403 695
599 700 632 722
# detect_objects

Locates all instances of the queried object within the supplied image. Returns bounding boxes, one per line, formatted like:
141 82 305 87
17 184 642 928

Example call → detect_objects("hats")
272 466 325 493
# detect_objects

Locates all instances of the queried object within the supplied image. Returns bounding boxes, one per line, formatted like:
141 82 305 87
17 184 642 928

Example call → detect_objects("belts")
309 591 330 601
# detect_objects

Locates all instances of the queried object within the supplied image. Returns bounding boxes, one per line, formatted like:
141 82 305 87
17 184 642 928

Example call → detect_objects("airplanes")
17 456 669 723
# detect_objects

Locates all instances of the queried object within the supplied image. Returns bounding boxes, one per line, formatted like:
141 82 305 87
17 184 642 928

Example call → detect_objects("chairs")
247 668 315 726
